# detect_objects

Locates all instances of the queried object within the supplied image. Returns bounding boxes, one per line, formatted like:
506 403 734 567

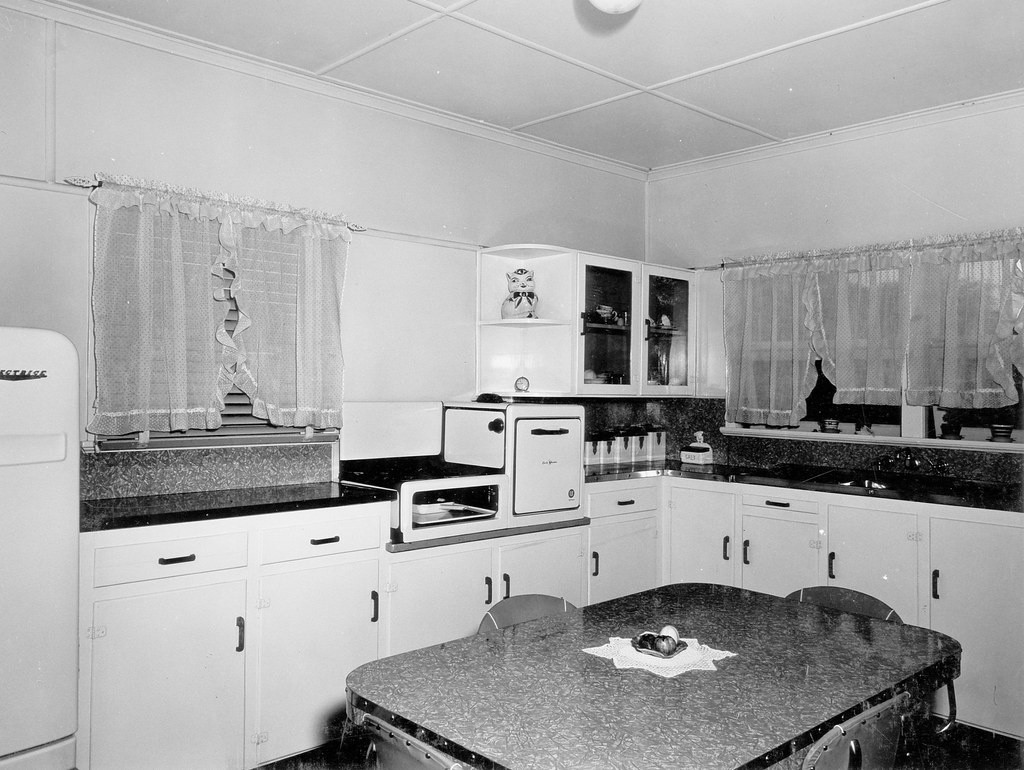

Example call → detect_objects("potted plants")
940 410 965 440
816 390 850 430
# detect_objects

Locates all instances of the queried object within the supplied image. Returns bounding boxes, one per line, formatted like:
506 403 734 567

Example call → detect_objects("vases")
989 425 1013 442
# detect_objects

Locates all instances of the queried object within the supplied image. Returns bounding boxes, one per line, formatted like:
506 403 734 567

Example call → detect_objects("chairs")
802 692 910 770
785 586 904 624
477 594 577 633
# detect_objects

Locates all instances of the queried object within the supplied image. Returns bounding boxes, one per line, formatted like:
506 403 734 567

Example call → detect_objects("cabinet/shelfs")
664 476 1024 741
76 498 250 770
583 477 663 606
385 525 589 658
475 243 697 398
251 501 389 770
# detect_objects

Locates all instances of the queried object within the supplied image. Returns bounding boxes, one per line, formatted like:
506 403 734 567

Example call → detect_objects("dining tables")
346 583 962 770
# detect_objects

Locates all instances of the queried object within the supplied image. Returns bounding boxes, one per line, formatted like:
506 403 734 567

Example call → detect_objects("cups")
622 312 631 326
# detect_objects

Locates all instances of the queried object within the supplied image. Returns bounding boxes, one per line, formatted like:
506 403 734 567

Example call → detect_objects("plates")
636 632 688 659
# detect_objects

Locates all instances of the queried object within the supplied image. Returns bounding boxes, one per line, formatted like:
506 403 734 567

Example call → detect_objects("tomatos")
654 635 676 655
